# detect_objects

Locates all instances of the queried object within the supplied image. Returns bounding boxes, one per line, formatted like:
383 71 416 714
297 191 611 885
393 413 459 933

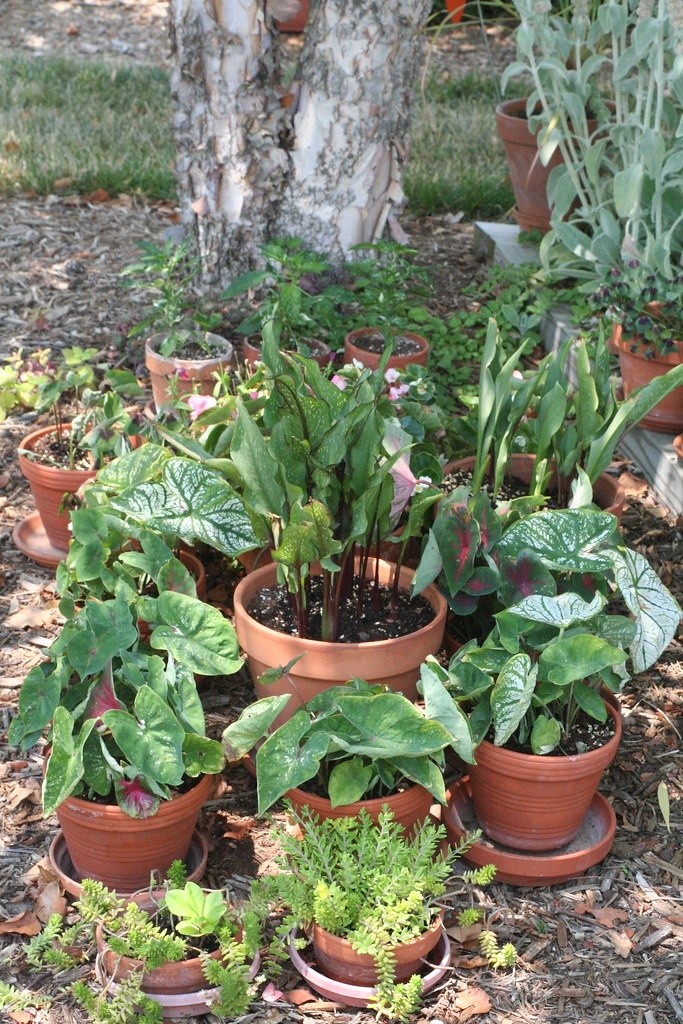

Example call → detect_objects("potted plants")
0 0 683 1024
